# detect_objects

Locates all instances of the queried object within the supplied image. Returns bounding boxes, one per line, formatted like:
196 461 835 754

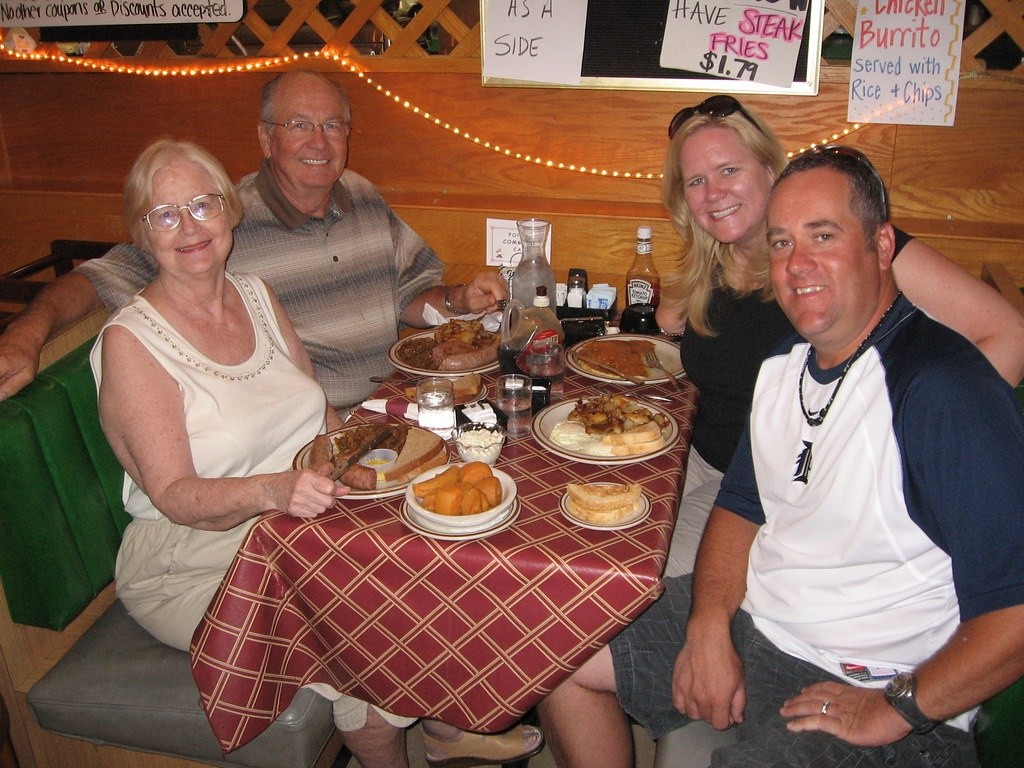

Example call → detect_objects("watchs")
883 671 936 735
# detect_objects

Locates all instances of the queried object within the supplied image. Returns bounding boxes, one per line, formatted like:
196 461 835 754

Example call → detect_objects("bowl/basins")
619 302 662 335
406 462 517 526
455 423 505 468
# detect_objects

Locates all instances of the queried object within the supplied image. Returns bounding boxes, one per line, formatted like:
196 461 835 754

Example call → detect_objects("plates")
292 423 451 500
417 376 490 406
399 495 522 541
559 482 653 530
531 396 681 465
564 334 688 385
388 329 500 377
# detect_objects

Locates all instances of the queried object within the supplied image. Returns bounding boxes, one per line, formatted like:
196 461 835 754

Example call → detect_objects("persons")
655 94 1024 581
0 67 512 426
533 144 1024 768
87 142 542 768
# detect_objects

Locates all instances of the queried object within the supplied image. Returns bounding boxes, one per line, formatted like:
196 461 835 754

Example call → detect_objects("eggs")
550 420 617 457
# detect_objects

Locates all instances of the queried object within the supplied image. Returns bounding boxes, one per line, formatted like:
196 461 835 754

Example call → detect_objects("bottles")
497 285 568 379
511 218 557 318
625 225 661 305
567 276 586 308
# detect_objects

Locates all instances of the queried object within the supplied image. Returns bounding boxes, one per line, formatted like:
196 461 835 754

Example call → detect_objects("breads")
404 372 482 398
384 428 449 483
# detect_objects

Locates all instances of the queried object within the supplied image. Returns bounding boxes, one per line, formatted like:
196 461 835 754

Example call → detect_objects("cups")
416 377 456 440
495 373 532 441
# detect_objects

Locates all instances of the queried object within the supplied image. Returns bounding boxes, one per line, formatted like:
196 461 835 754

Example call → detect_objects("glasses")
794 144 888 221
667 94 768 143
262 120 351 140
142 193 225 232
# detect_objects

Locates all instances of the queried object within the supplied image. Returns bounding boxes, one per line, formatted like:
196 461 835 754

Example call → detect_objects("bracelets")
445 283 467 316
658 328 687 339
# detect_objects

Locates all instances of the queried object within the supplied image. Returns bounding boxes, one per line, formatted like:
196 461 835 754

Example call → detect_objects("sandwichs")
567 482 641 524
602 421 665 456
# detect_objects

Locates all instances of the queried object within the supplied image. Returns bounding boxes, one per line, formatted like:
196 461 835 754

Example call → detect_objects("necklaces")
800 291 902 426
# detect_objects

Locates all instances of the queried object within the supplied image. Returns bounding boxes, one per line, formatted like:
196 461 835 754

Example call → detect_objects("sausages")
310 433 377 490
432 341 499 371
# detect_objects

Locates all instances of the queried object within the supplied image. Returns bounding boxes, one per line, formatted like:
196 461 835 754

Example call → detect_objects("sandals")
420 721 545 768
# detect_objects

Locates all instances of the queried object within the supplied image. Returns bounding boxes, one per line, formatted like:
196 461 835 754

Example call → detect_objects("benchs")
0 309 343 766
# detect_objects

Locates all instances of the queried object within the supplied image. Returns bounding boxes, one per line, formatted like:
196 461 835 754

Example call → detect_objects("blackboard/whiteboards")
478 0 825 96
0 0 244 27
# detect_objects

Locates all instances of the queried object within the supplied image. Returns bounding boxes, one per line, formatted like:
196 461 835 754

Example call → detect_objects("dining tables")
188 314 699 756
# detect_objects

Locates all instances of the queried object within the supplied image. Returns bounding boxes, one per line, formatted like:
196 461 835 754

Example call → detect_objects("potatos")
589 396 665 429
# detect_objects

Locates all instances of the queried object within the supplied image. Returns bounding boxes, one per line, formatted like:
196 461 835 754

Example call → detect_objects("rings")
821 701 829 715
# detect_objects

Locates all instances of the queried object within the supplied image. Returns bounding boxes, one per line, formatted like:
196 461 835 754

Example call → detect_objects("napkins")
421 301 504 331
360 397 418 422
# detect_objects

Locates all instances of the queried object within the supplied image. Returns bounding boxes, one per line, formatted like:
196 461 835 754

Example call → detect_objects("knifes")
566 345 644 385
325 430 394 481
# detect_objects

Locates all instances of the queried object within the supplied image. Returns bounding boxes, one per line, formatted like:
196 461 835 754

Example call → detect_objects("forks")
644 347 684 392
495 299 506 309
